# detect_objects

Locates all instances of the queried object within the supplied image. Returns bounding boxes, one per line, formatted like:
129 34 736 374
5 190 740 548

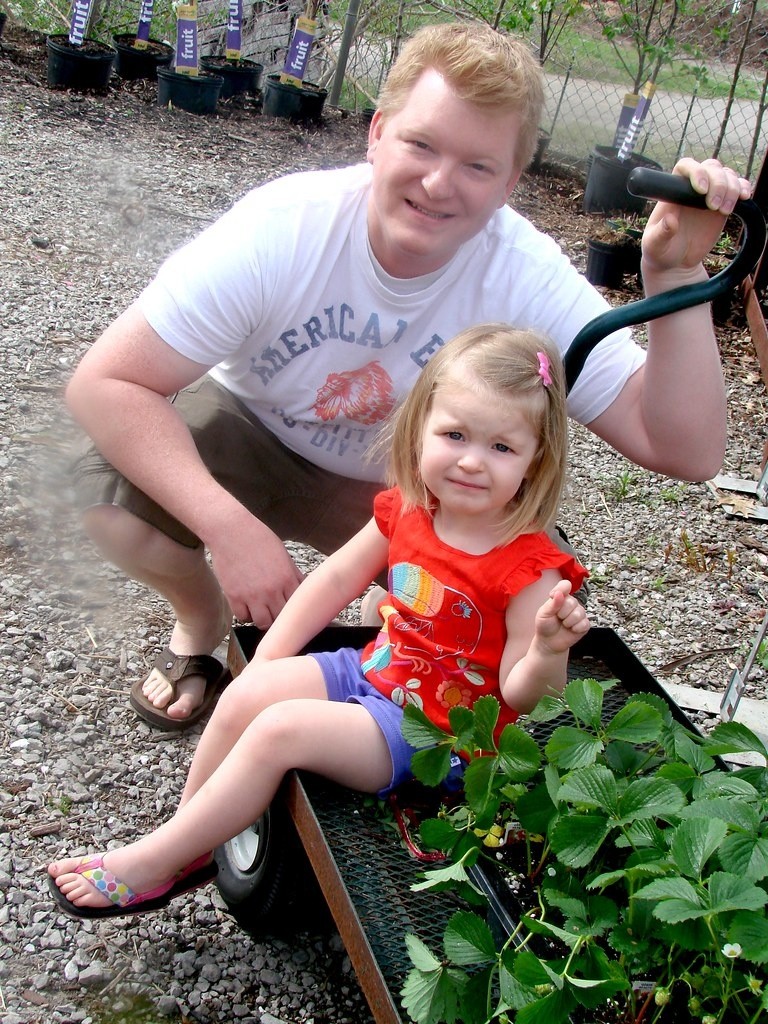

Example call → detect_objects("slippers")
130 642 230 734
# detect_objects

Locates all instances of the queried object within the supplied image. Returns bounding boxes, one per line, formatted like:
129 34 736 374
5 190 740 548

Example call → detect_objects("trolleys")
211 142 765 1024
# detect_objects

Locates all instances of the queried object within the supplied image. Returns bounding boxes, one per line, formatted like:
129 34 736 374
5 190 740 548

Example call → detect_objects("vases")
111 33 176 82
44 31 117 92
155 65 224 115
262 72 328 121
582 145 663 216
528 128 553 174
200 54 264 98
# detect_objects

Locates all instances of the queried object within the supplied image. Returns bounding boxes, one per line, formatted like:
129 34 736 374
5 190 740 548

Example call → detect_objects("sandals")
47 842 176 919
172 849 220 903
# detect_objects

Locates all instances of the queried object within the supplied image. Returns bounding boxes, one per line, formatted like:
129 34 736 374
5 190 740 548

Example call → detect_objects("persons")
62 20 751 732
48 321 589 918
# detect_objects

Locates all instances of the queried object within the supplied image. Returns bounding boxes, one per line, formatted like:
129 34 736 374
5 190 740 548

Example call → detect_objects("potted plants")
703 253 734 325
398 677 768 1024
605 213 648 244
710 231 736 259
586 239 630 289
